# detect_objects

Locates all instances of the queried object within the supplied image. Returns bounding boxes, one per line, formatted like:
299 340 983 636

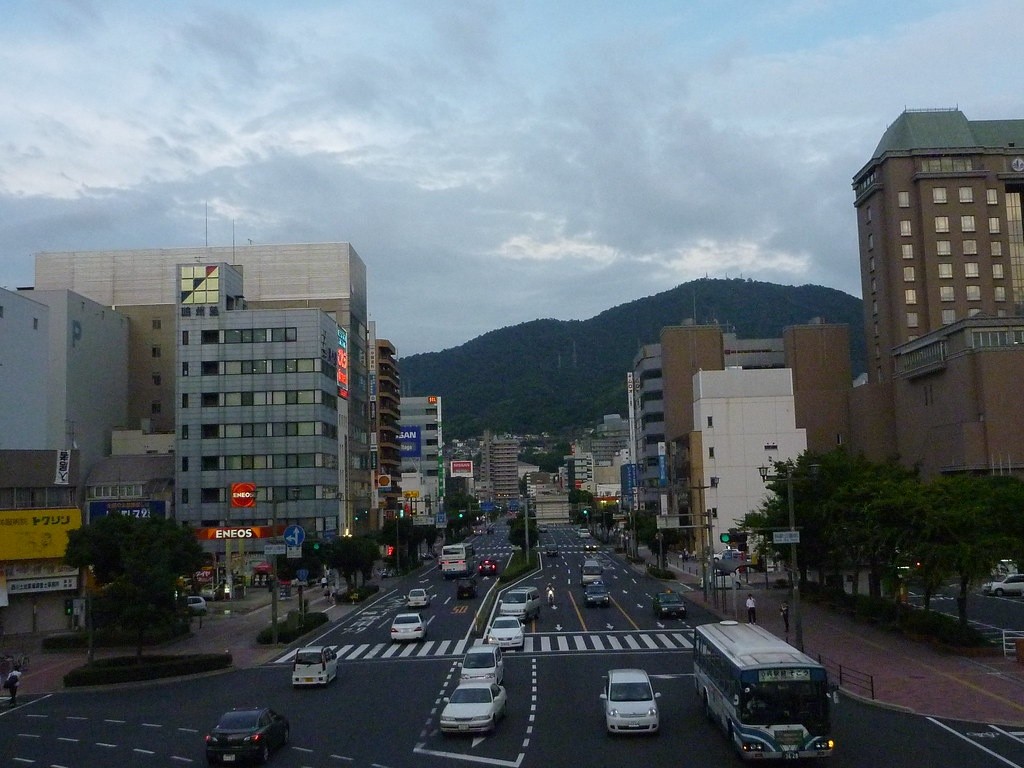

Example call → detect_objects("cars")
653 591 687 619
699 574 741 589
188 596 208 613
600 669 662 735
200 584 221 600
205 707 290 767
391 613 428 642
293 646 338 686
579 559 610 608
546 547 560 557
406 589 432 607
489 617 526 650
577 529 592 539
440 680 508 733
584 541 597 551
538 524 549 534
479 560 500 576
454 577 479 598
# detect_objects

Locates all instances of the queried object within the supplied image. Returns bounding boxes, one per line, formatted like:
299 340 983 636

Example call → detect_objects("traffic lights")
583 510 588 514
458 512 466 521
311 540 322 553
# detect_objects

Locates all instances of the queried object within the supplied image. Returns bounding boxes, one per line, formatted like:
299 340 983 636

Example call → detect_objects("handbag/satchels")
3 675 18 688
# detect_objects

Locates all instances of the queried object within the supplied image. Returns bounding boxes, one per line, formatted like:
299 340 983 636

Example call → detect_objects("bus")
694 621 835 764
441 544 475 579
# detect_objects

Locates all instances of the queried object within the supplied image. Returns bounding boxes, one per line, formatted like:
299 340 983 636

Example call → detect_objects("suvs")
707 548 738 562
981 572 1024 597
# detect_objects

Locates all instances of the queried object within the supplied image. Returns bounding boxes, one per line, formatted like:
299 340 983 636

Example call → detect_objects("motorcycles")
546 590 556 607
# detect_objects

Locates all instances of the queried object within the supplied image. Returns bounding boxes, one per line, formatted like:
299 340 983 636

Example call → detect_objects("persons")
746 593 757 623
546 583 554 592
747 692 765 712
780 601 789 632
6 666 21 707
324 583 335 602
682 548 688 562
321 575 327 590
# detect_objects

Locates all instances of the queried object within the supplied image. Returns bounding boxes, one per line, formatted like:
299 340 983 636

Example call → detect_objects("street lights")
758 462 821 652
719 533 748 543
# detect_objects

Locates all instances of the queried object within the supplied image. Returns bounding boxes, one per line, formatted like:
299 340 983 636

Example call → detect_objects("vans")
457 645 506 685
498 586 544 622
